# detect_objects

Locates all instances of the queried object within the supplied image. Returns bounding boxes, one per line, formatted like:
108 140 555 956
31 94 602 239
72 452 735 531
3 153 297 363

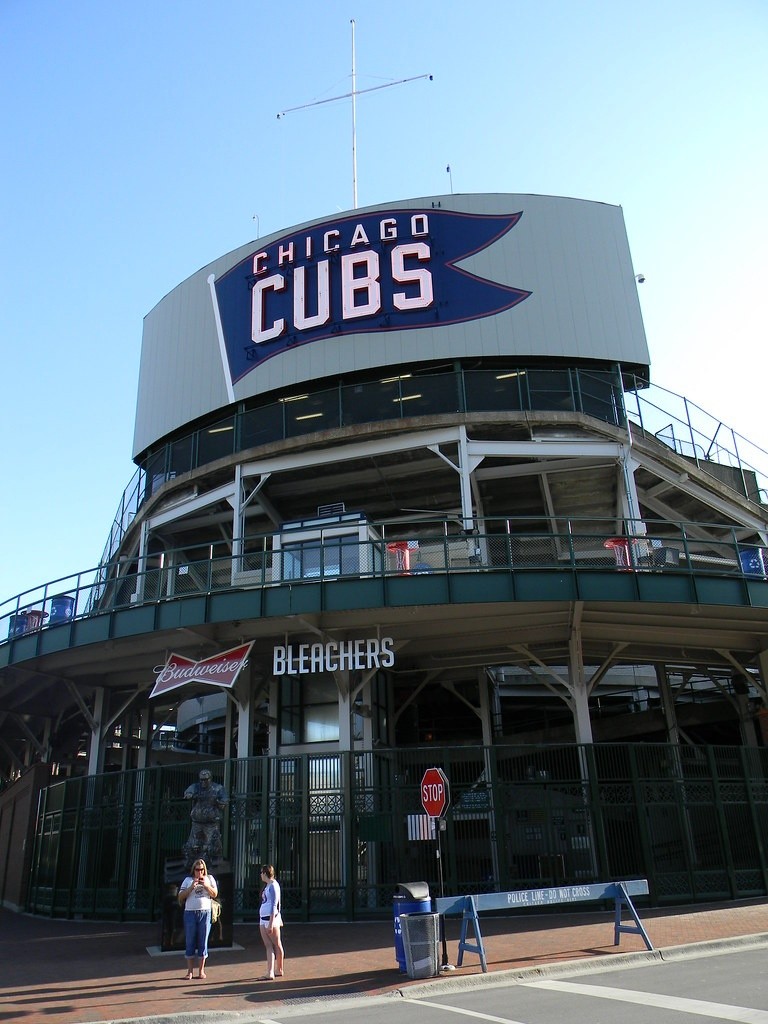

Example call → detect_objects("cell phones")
198 878 203 884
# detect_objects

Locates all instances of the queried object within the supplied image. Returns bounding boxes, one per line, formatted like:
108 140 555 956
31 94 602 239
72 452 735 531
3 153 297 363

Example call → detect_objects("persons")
181 770 227 869
259 864 284 981
178 858 218 979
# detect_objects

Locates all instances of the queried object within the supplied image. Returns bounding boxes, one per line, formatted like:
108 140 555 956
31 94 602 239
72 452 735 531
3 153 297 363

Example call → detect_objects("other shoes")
185 970 193 979
255 976 274 981
199 973 206 979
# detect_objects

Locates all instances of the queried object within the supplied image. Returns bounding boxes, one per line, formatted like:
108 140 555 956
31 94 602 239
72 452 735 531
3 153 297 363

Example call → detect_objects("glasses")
195 868 204 872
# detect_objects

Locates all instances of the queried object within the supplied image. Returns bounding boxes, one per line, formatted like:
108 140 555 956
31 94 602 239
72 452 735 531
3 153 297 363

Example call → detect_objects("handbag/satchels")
211 898 221 923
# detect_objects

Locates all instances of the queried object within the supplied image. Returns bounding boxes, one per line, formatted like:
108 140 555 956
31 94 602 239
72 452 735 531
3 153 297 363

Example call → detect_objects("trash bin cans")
392 881 432 973
398 911 440 979
